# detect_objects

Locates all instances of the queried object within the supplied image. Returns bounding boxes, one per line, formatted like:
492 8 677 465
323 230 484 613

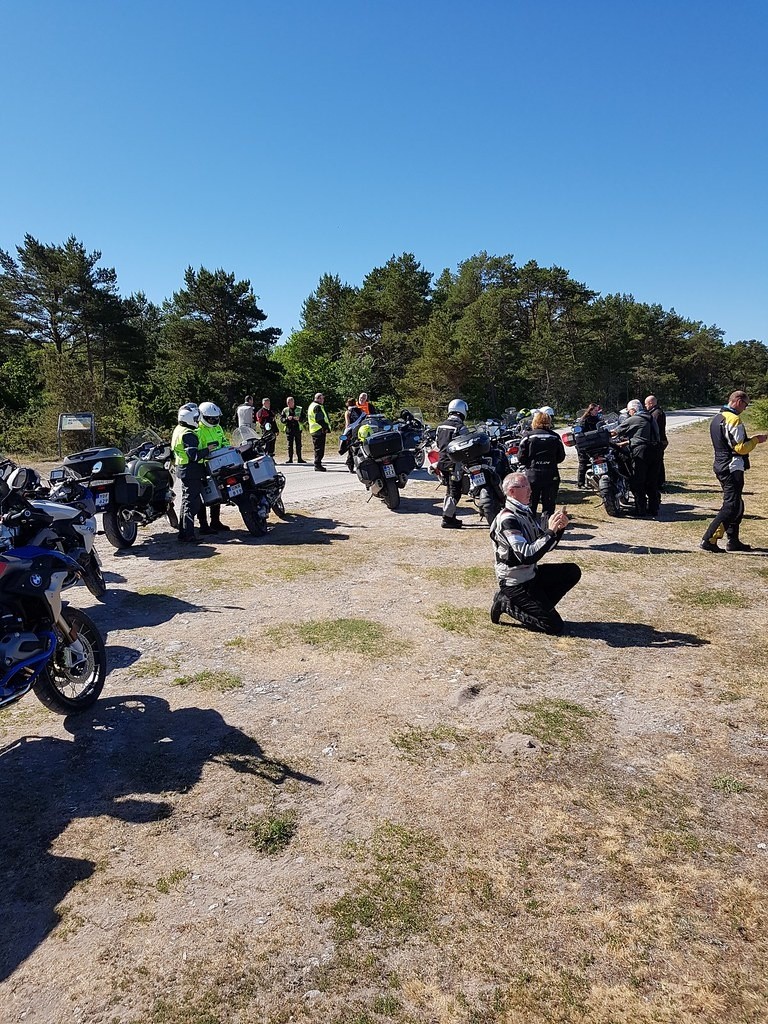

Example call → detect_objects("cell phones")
561 506 566 514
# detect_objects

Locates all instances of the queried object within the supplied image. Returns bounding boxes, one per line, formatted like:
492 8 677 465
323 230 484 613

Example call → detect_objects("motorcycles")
339 406 633 524
63 440 180 549
1 456 107 717
205 432 287 535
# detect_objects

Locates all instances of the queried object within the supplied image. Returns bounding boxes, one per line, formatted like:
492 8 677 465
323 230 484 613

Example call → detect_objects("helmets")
6 467 41 498
178 403 200 429
199 402 222 427
541 406 554 419
515 408 531 422
401 409 410 419
447 399 469 418
357 424 374 442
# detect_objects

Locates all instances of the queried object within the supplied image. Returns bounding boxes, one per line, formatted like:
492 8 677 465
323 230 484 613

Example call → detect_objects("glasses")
512 483 531 488
736 398 748 408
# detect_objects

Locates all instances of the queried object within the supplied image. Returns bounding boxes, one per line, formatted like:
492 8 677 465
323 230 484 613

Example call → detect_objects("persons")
490 472 582 637
515 406 567 515
435 399 469 528
256 397 279 466
698 391 768 553
610 395 668 519
574 403 606 489
280 397 306 463
171 401 230 543
345 392 376 473
308 393 332 472
234 395 254 427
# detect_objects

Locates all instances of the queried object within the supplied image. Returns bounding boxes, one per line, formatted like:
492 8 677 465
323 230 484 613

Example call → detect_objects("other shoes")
178 535 183 541
314 466 326 471
182 536 204 543
200 526 218 534
211 522 230 531
441 516 462 529
298 459 307 463
286 460 293 463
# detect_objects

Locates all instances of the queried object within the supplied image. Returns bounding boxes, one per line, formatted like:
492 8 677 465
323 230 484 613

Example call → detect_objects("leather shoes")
700 540 726 553
727 539 751 550
490 591 507 623
